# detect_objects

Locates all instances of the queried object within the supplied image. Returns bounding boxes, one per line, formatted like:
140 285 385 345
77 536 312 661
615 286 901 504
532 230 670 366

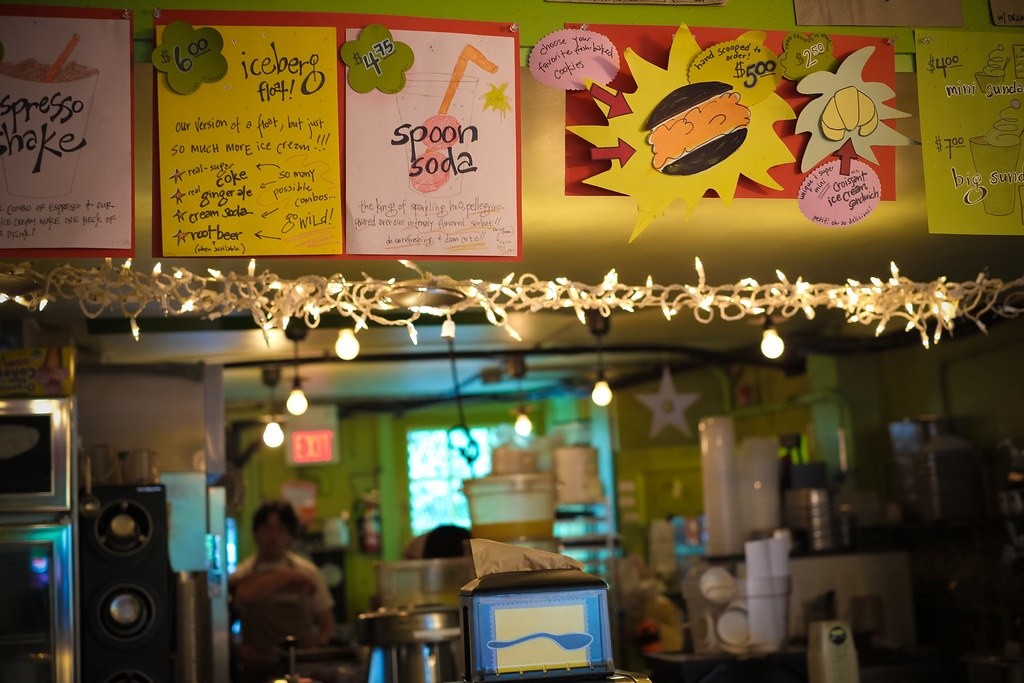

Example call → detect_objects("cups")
700 415 790 651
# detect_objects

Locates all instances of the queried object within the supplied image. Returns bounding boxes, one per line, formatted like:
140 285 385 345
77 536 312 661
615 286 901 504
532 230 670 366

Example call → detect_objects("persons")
401 525 471 683
229 499 335 648
